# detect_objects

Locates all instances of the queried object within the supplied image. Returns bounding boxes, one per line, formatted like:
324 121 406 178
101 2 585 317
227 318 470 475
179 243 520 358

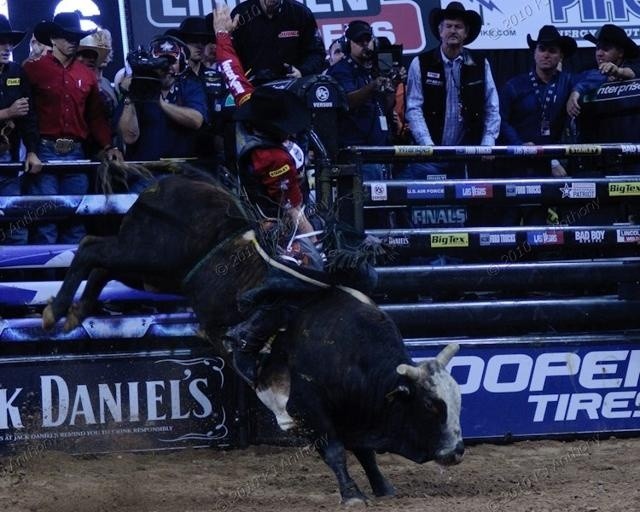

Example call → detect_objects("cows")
43 175 466 508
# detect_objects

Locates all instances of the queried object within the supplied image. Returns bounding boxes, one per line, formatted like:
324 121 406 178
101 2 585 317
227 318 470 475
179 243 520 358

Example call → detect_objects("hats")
178 17 214 40
584 25 639 57
428 1 484 47
0 13 28 50
33 13 97 44
232 85 311 141
75 36 113 66
370 37 403 59
149 37 181 62
527 25 578 60
345 19 373 42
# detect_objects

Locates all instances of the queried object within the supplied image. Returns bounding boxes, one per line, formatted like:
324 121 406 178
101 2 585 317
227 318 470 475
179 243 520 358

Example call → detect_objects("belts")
38 135 83 156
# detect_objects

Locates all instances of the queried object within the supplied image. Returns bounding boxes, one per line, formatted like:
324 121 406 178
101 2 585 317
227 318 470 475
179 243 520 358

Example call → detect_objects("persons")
393 82 408 137
180 15 211 83
116 36 210 162
76 37 113 123
23 10 123 245
1 14 42 245
233 1 326 80
408 0 502 180
326 21 408 180
596 25 640 81
212 2 325 387
323 38 345 73
89 29 116 104
501 25 605 179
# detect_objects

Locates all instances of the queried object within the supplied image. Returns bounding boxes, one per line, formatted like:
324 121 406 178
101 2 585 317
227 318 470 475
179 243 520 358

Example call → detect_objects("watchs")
104 144 119 152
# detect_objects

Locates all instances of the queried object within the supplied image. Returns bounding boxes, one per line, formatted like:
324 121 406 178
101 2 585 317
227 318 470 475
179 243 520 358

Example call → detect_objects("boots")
221 292 302 389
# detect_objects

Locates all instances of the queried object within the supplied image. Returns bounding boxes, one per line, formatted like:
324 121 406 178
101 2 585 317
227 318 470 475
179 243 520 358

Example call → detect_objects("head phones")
340 19 378 55
148 35 189 78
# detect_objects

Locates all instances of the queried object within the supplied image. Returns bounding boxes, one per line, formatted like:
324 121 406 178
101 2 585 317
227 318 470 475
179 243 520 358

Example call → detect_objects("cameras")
126 51 170 103
374 37 403 83
